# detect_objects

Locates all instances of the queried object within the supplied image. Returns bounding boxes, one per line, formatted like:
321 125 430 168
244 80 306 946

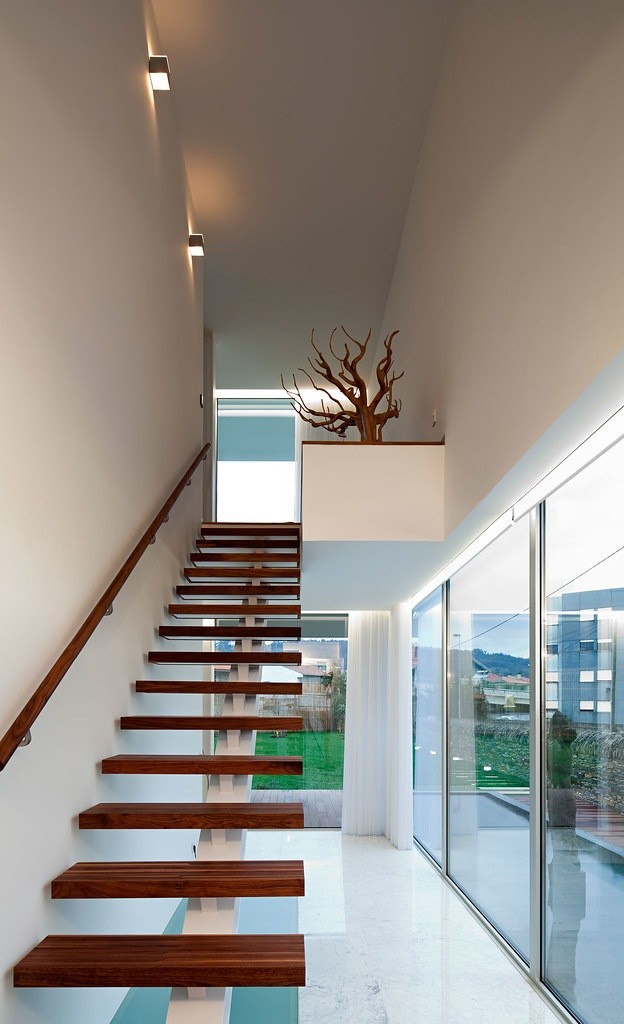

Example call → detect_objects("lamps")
150 55 172 90
190 234 205 256
